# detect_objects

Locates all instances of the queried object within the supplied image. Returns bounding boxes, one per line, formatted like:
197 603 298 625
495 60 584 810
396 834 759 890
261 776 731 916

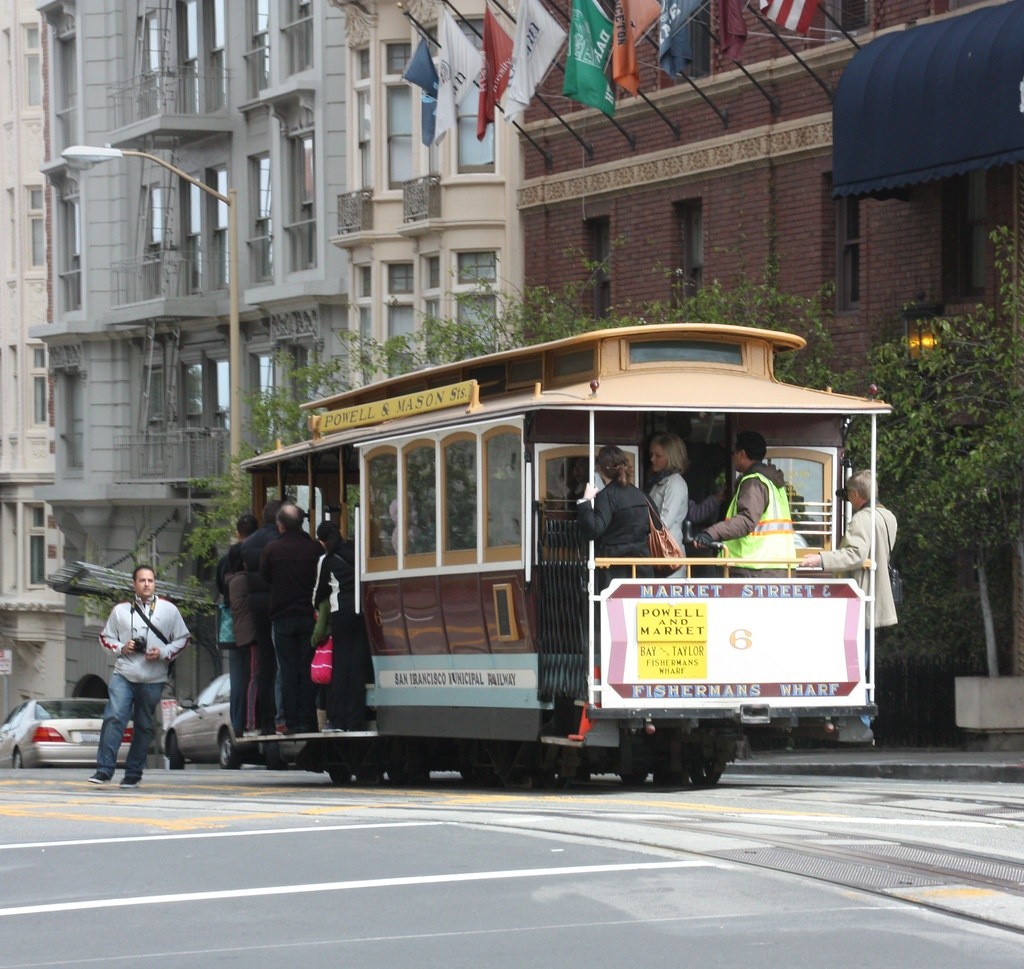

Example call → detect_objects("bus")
236 323 893 789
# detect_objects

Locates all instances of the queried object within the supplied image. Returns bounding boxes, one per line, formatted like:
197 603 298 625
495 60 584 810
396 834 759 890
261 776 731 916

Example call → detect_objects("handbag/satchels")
217 602 236 650
642 494 684 578
311 636 334 685
888 565 903 606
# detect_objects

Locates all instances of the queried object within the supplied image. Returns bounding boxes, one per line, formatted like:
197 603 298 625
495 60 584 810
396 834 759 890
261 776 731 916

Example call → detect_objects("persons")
370 456 589 559
88 565 191 788
215 499 374 737
576 430 897 728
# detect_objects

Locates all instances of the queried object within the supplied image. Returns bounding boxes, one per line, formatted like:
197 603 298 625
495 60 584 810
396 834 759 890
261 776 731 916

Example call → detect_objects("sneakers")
89 772 110 784
119 777 139 788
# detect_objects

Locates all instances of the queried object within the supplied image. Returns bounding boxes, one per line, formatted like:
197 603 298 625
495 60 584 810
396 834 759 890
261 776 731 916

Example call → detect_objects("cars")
162 673 306 770
0 698 134 768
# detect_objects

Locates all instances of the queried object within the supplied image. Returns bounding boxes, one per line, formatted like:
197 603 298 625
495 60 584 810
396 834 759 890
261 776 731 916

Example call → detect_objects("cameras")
131 636 147 653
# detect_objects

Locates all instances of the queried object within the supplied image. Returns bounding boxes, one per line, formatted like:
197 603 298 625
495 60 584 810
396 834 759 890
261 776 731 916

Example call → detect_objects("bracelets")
577 499 586 505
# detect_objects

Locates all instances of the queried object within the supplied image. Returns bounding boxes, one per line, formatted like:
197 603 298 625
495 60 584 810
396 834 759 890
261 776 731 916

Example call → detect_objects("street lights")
59 142 240 546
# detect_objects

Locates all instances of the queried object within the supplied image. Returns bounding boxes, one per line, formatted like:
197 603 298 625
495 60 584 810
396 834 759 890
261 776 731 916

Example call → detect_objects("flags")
761 0 820 35
477 8 513 142
563 0 615 116
503 0 566 126
400 38 438 147
658 0 702 79
719 0 746 60
434 8 482 148
612 0 660 99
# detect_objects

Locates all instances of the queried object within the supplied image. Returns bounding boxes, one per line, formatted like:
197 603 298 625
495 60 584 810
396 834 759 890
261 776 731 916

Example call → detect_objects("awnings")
830 0 1024 197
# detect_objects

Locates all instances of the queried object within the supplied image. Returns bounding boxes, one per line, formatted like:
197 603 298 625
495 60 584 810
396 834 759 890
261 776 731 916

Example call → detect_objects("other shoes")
322 722 343 732
276 725 289 735
